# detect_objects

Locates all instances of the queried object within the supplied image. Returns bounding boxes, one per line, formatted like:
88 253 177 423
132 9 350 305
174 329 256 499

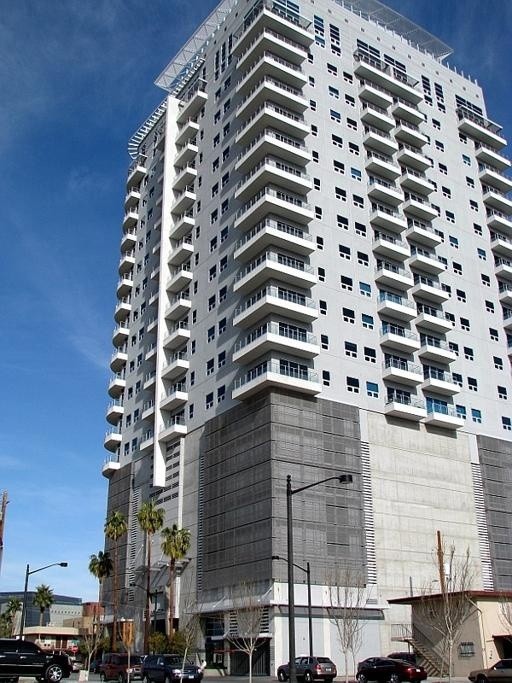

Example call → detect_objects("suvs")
0 637 204 683
277 655 338 682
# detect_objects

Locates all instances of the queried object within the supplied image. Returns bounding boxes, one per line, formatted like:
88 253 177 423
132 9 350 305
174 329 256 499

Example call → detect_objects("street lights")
270 554 314 658
129 581 159 655
285 472 354 683
20 561 69 640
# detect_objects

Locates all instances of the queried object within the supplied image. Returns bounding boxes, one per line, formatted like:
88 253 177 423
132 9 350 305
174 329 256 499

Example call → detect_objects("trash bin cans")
78 670 88 681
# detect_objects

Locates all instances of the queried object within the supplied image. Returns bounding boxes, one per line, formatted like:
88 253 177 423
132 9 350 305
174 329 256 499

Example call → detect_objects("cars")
467 657 512 683
354 650 427 682
